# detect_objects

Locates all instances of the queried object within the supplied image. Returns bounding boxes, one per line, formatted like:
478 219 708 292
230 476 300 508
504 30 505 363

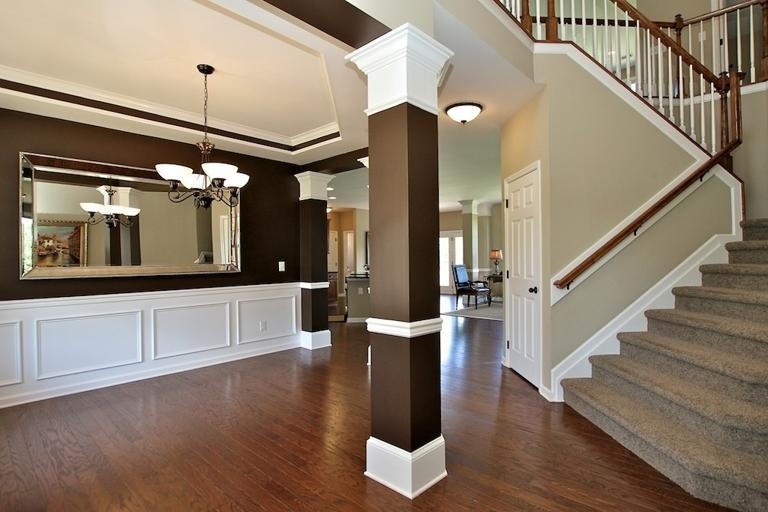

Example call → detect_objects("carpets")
440 301 504 322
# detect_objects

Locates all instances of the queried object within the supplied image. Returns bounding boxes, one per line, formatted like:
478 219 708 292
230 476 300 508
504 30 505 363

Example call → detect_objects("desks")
484 274 503 297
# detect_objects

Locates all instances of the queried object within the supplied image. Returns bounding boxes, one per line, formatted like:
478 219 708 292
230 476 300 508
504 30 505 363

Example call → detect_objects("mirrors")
19 152 243 280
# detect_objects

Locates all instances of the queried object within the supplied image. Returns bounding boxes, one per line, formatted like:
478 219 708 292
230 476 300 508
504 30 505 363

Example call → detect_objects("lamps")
79 185 141 230
154 63 250 211
489 249 504 275
444 101 484 125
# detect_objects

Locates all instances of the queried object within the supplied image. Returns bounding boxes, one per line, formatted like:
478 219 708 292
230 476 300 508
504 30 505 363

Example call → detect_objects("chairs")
451 264 492 310
